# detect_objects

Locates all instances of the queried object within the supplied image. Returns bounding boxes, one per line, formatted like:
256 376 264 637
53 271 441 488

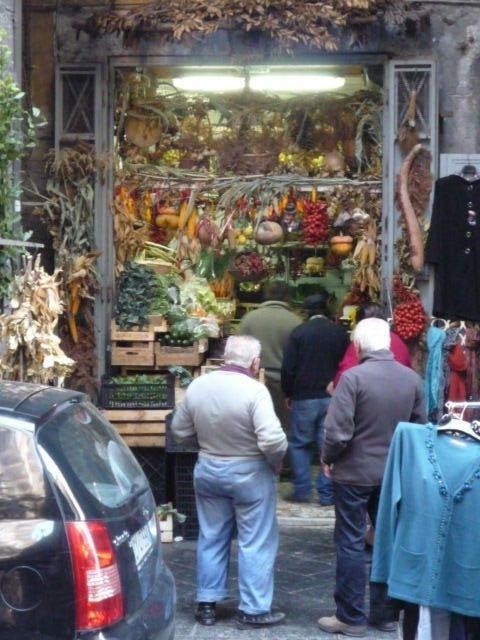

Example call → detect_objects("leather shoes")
194 605 217 626
317 615 399 637
236 609 286 631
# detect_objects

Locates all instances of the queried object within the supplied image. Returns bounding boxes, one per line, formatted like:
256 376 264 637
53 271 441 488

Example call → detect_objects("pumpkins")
255 221 283 244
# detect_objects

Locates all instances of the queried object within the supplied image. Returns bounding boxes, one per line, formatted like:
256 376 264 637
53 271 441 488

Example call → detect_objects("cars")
0 378 178 640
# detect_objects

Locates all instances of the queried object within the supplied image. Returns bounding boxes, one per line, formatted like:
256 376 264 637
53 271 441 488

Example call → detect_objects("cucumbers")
158 319 208 346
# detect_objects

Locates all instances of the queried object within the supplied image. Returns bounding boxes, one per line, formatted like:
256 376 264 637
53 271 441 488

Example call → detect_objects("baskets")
99 374 176 411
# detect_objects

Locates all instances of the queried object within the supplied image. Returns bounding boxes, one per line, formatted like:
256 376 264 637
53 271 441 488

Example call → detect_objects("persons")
316 316 429 637
237 277 304 482
169 333 292 629
280 292 351 507
324 303 412 395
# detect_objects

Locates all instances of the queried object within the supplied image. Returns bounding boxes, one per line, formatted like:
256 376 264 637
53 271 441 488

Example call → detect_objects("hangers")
426 403 479 445
441 150 480 184
